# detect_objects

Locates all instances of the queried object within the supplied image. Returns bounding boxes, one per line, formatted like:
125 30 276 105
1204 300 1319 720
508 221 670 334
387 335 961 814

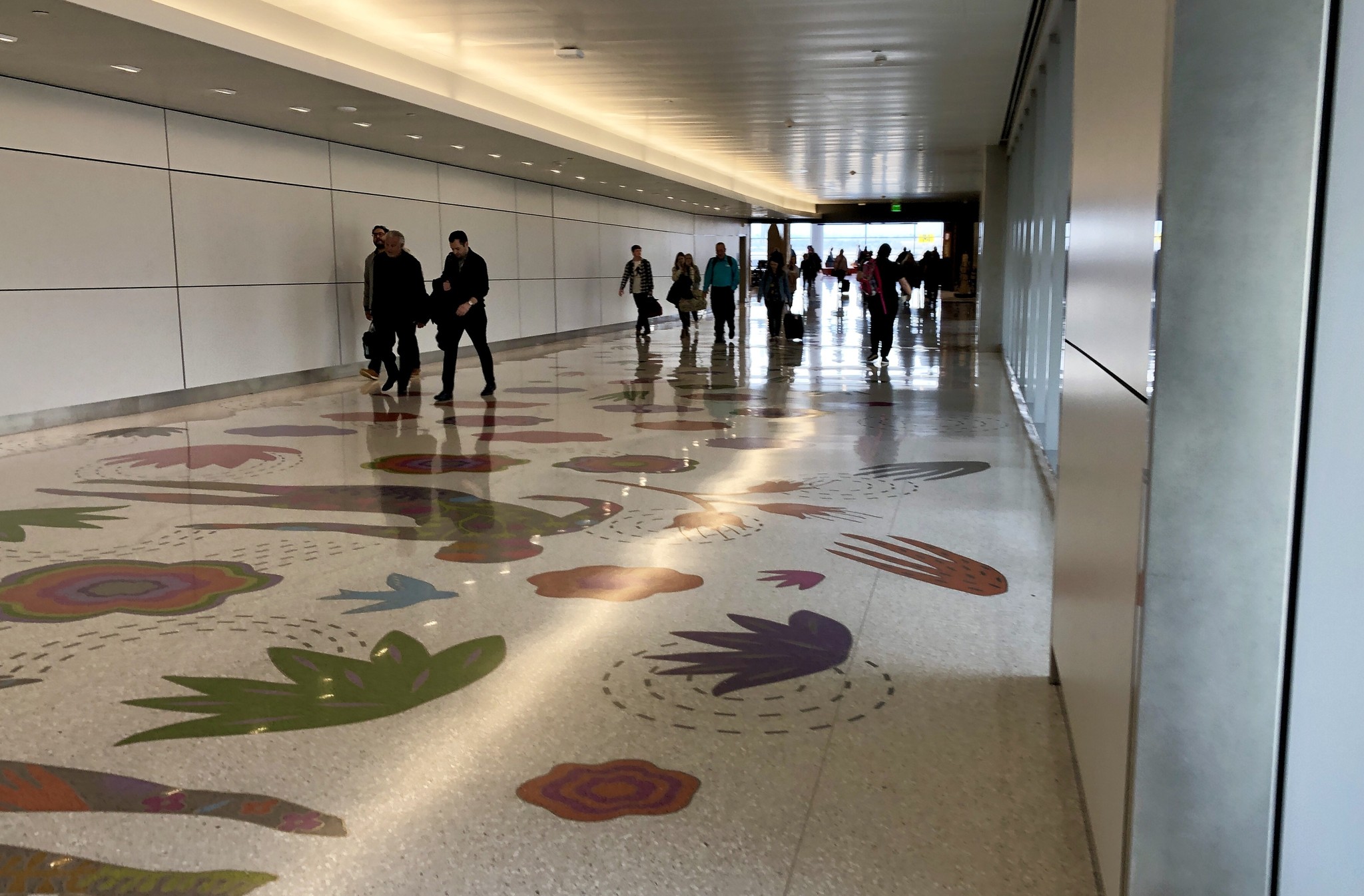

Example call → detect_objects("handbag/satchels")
415 293 432 327
678 288 707 311
362 320 377 360
640 294 663 318
859 257 887 314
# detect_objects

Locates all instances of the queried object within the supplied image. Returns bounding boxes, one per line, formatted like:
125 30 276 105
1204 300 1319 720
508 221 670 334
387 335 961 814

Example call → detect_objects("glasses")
373 232 384 237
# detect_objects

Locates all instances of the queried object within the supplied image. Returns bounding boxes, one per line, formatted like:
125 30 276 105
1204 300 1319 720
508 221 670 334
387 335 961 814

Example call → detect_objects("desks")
939 289 977 348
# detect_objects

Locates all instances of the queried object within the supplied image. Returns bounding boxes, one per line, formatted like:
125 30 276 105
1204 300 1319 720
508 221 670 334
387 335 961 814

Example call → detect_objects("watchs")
467 301 474 306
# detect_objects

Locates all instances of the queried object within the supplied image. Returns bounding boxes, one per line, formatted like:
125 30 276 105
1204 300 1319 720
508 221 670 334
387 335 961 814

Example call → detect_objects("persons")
619 245 654 344
434 231 497 401
789 243 959 364
684 253 702 330
672 251 692 338
702 242 741 344
758 251 792 341
359 225 421 380
368 231 423 396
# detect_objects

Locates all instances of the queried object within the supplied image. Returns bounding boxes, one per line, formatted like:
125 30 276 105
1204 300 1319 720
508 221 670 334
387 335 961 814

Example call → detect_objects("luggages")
782 301 805 340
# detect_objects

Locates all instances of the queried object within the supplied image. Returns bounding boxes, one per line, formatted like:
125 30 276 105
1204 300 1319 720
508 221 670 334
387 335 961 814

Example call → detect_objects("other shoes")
397 381 407 396
410 367 421 378
480 382 496 396
866 353 878 361
433 390 453 401
881 357 889 365
382 371 399 392
359 367 380 380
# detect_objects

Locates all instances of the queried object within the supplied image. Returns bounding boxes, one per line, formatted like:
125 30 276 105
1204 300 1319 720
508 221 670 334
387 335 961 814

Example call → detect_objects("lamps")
552 46 583 60
